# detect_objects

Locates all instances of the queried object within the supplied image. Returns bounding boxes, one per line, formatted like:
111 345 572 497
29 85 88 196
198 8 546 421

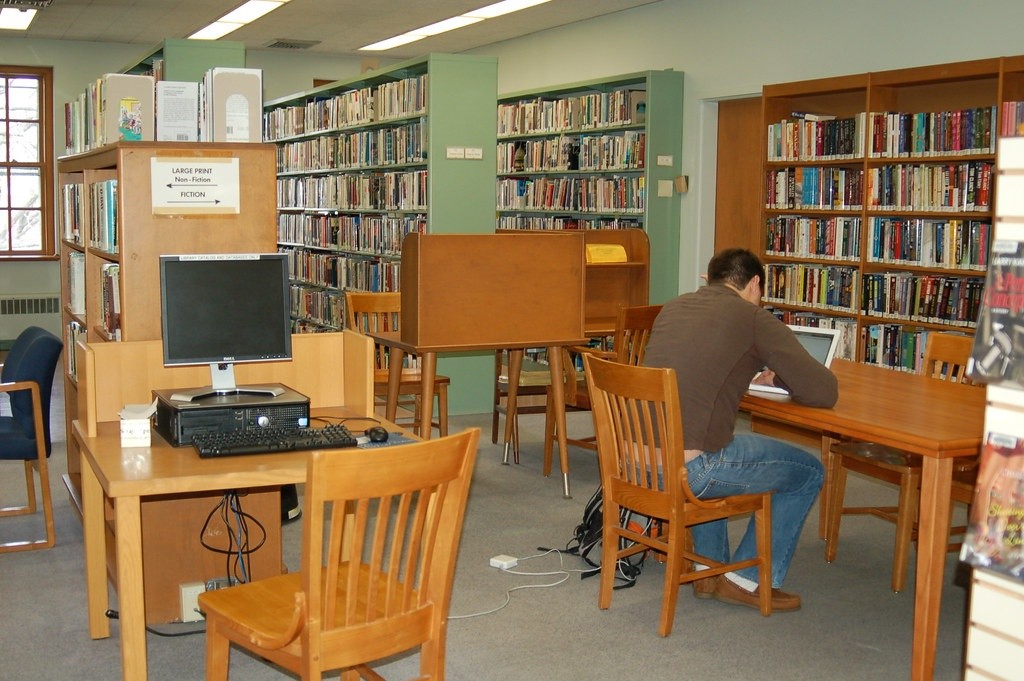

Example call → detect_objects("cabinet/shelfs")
751 56 1023 448
262 52 500 418
497 69 685 385
58 141 282 625
113 36 246 84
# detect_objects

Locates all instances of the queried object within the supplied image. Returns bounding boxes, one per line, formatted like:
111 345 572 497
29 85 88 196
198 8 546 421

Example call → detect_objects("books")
521 338 632 371
770 99 1024 384
64 66 261 155
251 75 428 370
960 432 1023 581
496 91 648 229
57 178 122 378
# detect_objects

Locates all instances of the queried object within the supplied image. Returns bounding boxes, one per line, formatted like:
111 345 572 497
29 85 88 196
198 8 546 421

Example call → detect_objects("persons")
622 247 840 611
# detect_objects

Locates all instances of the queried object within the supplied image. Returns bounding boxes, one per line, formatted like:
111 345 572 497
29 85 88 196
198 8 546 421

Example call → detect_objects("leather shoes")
713 575 801 611
693 576 715 599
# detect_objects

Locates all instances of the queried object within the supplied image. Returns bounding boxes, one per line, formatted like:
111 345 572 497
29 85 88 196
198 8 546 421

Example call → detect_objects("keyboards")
192 424 358 458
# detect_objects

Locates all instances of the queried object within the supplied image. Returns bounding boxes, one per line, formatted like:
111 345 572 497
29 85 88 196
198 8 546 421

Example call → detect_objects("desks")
72 329 429 681
739 358 986 681
363 229 651 498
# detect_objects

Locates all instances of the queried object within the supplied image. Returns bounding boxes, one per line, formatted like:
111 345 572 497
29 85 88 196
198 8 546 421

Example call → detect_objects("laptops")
749 325 841 394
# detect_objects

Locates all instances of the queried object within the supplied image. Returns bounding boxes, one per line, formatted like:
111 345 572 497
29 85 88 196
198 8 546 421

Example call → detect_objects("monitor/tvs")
159 253 294 402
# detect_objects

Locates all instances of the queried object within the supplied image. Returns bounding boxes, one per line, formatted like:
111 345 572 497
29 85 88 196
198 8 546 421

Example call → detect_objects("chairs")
345 292 450 439
543 306 664 477
0 326 64 554
825 332 981 594
581 351 772 637
197 428 483 681
491 349 585 444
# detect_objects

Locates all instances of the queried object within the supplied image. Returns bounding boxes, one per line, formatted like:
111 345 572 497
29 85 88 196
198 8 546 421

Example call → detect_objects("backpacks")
574 484 651 589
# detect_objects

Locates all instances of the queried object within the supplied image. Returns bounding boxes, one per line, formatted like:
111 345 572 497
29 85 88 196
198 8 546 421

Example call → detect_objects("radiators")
0 293 62 340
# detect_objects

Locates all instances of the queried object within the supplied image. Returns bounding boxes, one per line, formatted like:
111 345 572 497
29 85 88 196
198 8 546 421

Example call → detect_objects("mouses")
365 425 388 442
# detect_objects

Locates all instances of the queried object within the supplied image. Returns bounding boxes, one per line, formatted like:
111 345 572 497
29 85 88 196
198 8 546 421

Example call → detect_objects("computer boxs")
151 382 311 448
